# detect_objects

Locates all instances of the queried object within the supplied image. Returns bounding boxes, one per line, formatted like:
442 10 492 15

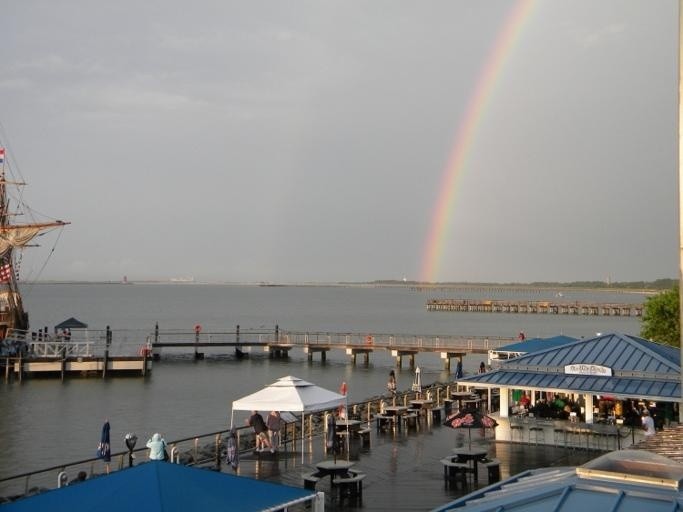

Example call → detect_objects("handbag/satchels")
164 449 169 460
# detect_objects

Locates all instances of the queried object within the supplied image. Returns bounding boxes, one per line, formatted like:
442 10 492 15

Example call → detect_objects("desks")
453 393 471 412
453 448 487 480
316 460 354 488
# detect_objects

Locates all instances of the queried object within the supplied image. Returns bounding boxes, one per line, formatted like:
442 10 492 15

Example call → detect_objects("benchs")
440 455 501 492
303 471 365 512
335 397 481 447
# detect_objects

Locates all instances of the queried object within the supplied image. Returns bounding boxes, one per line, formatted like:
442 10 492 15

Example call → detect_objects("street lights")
125 431 137 468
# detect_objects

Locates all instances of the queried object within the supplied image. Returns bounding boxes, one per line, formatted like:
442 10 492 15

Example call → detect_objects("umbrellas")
478 361 485 373
327 414 339 464
96 419 112 474
338 382 350 420
411 366 423 394
455 359 463 379
388 370 398 394
443 407 499 448
224 425 239 473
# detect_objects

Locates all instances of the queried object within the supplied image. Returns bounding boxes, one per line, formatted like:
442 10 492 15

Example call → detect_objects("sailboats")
0 148 72 366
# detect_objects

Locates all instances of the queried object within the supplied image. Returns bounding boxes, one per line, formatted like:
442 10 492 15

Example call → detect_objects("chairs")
507 413 618 457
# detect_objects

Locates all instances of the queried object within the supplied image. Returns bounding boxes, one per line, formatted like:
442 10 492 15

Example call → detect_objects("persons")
245 411 276 454
519 331 525 342
145 433 168 461
266 411 281 448
559 404 580 419
78 471 87 482
640 409 656 436
